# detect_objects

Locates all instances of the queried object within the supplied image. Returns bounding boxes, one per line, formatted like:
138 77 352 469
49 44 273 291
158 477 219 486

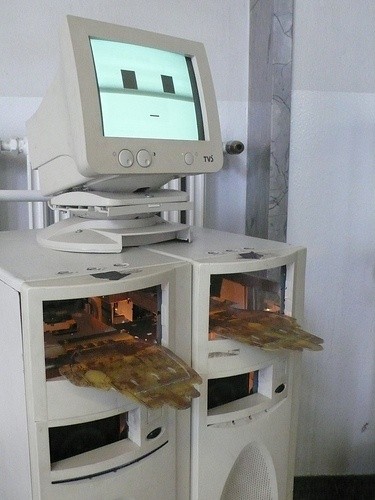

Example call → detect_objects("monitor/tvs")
24 15 223 195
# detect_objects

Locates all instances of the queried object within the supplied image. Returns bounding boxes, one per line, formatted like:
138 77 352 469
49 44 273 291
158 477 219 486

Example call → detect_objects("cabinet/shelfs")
143 225 308 500
0 228 192 500
